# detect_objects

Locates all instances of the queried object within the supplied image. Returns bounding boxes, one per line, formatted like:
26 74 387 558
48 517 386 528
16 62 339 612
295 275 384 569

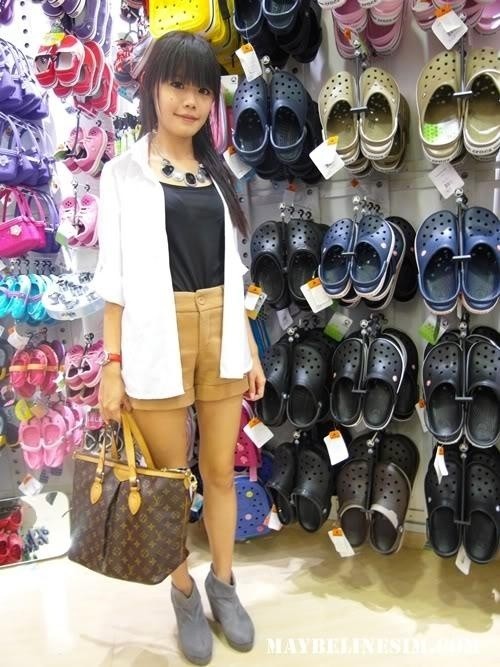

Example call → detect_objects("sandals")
0 273 61 326
0 340 83 471
0 500 51 565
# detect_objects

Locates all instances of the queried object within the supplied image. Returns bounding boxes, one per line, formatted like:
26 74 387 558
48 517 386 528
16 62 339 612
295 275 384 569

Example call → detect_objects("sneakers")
33 29 117 119
63 194 100 248
118 31 152 101
61 125 113 178
40 1 112 56
79 340 107 430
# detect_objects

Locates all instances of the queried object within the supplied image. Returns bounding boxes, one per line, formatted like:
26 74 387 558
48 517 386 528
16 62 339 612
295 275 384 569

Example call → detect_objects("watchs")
97 351 122 366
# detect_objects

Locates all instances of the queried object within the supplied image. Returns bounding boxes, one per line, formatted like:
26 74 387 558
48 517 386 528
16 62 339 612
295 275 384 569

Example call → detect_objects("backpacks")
231 476 277 542
235 397 262 469
148 0 231 50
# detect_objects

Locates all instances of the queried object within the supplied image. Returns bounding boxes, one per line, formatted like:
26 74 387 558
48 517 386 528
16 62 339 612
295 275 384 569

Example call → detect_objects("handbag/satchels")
1 188 59 257
64 409 193 584
0 40 48 118
0 110 53 186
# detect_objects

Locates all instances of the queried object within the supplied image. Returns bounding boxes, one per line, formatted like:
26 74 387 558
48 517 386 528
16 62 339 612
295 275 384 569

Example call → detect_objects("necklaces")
147 135 209 187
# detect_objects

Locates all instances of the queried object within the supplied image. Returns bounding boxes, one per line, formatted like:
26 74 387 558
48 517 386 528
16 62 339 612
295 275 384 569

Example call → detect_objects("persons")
92 29 266 665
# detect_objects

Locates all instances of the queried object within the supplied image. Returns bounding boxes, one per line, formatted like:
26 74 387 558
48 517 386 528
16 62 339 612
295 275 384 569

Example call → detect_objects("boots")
205 563 255 653
170 577 212 666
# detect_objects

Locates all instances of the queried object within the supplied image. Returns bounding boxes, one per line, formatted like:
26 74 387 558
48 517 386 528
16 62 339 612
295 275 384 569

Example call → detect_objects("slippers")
231 1 499 61
230 46 499 182
257 326 499 449
266 433 499 564
249 207 500 315
